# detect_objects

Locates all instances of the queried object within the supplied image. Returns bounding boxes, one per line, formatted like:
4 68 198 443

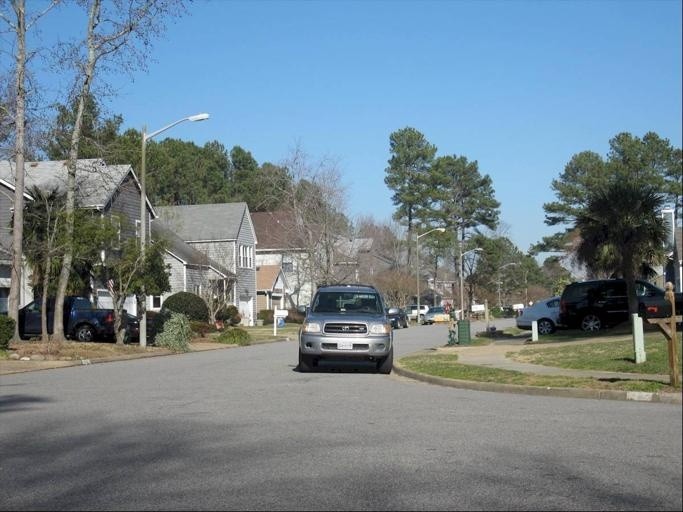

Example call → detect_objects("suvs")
298 283 393 374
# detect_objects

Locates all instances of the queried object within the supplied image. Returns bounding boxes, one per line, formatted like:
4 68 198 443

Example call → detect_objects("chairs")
362 301 376 311
323 299 337 311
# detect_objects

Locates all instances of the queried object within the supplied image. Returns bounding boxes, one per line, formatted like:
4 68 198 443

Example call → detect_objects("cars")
126 313 155 342
423 307 450 324
385 307 409 328
516 279 683 336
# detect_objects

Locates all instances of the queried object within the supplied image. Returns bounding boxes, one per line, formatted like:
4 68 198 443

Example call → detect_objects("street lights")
139 112 213 347
496 261 516 306
415 226 446 323
460 247 483 320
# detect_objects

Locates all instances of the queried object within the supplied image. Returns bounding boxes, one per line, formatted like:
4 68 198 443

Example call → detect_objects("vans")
403 304 429 321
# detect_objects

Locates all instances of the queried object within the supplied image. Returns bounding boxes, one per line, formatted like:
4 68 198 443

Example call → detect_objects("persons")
447 305 458 344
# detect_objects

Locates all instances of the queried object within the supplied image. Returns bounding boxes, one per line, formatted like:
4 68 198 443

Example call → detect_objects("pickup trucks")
0 296 127 342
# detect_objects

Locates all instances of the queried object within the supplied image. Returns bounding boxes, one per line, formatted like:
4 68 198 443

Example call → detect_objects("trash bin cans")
458 320 470 345
